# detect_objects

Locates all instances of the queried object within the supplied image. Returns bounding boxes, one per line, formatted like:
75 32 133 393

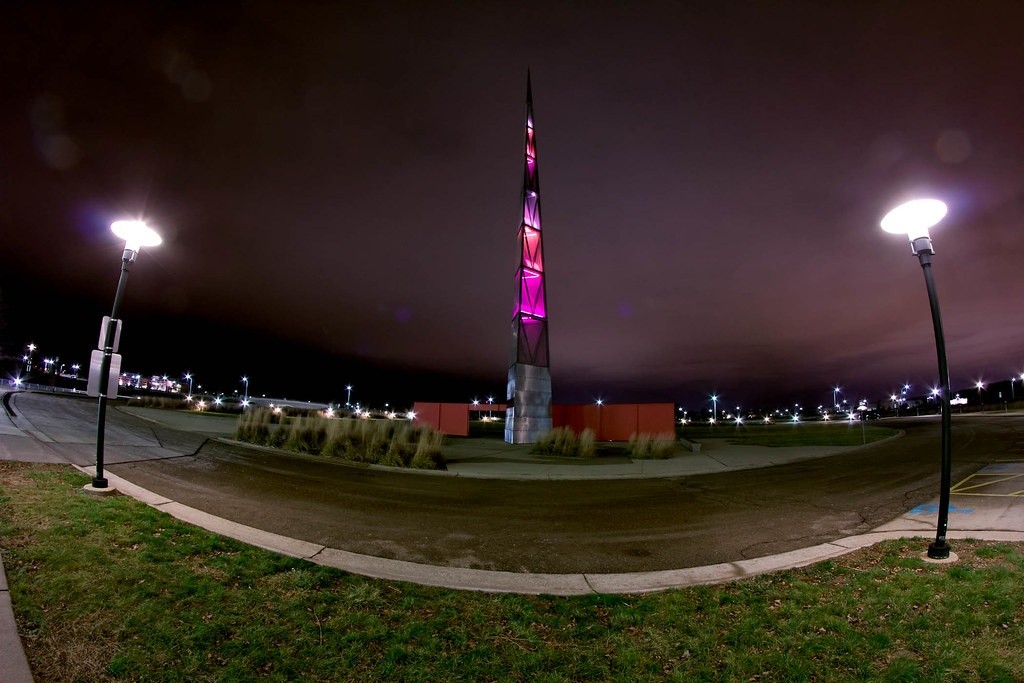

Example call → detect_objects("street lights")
489 398 493 417
1011 378 1015 399
186 375 192 393
833 387 839 407
347 385 351 409
881 198 954 558
712 395 716 422
86 220 163 487
242 377 248 396
977 382 982 404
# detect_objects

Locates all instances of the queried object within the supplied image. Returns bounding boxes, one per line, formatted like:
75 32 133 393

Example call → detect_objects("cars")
859 412 881 421
